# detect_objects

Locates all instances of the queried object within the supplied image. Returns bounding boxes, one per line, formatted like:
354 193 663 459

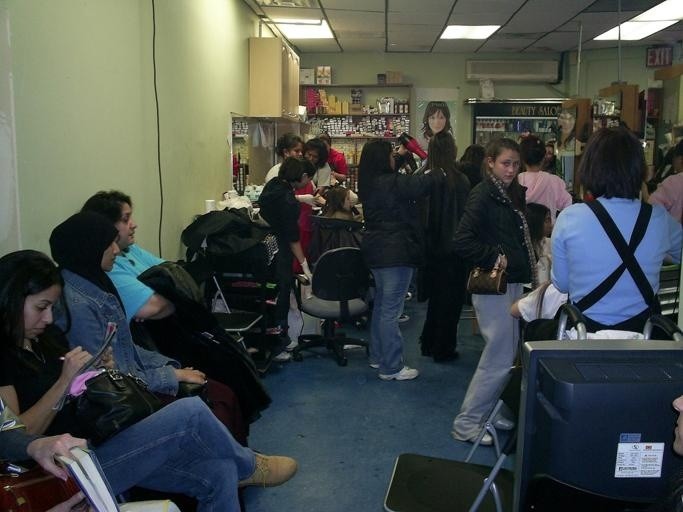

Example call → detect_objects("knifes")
464 58 563 85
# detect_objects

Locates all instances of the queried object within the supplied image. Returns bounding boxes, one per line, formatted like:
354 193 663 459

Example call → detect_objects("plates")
299 83 412 189
250 36 300 118
465 97 575 187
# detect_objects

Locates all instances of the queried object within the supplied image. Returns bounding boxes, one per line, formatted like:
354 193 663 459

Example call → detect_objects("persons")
641 136 682 226
673 395 682 453
454 136 538 446
84 190 273 424
523 200 554 290
51 213 250 443
551 125 683 343
0 393 93 512
458 144 486 187
409 132 471 362
317 133 347 183
322 183 363 222
302 138 331 197
517 130 572 222
421 101 452 140
552 100 583 157
511 283 569 320
260 156 312 363
0 249 299 512
265 133 304 184
358 138 446 382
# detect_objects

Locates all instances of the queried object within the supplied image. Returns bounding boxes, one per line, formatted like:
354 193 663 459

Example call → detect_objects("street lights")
70 371 162 440
467 263 508 294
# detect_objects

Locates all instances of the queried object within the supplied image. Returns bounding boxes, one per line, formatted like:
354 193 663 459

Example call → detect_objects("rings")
58 357 65 360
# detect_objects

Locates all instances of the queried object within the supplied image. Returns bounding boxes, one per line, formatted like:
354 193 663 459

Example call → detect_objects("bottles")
49 213 117 289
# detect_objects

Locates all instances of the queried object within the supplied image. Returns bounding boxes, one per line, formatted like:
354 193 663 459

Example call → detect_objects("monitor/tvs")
55 447 119 511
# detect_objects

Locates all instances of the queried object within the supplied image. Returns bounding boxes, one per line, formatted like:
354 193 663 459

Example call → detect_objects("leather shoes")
299 257 313 289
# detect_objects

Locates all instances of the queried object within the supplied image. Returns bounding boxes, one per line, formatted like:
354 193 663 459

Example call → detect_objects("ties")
513 340 682 512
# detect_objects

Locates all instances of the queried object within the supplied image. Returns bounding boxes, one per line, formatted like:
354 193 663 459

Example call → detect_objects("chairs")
493 413 518 433
369 361 379 369
238 452 297 489
453 429 494 446
378 367 419 380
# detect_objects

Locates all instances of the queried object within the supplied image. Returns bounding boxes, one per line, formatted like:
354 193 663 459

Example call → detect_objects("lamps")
658 264 680 323
292 247 374 366
193 264 271 377
385 317 557 512
